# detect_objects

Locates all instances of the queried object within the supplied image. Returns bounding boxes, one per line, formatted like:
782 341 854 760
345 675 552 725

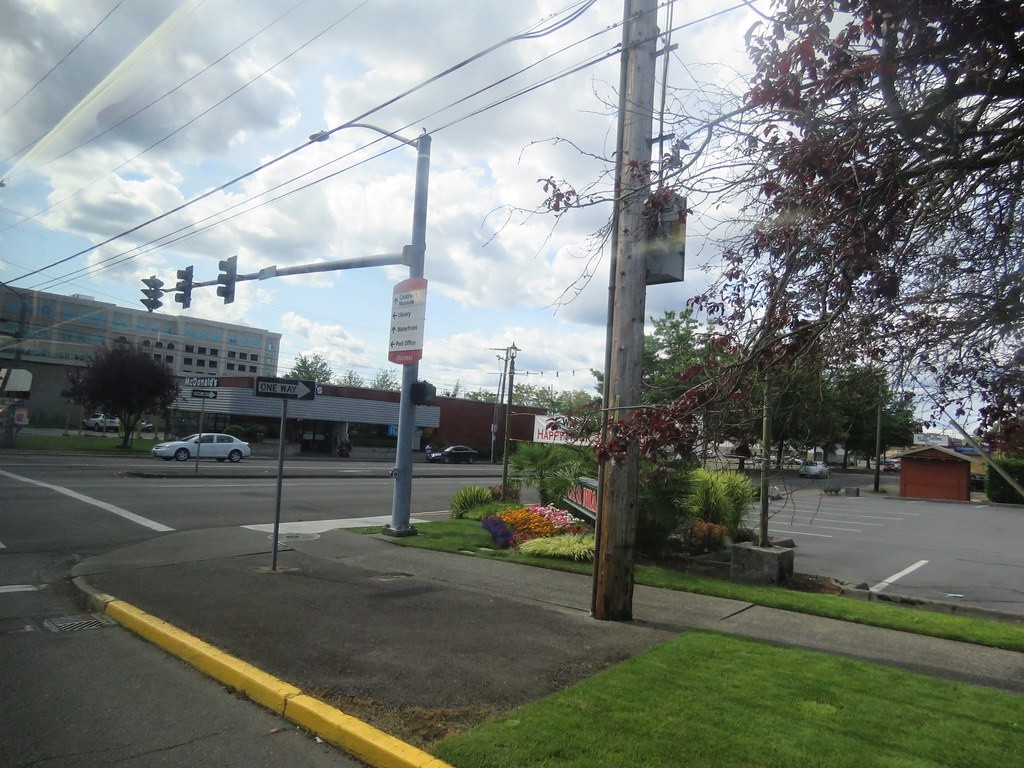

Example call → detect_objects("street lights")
873 372 887 493
498 341 521 501
309 122 433 530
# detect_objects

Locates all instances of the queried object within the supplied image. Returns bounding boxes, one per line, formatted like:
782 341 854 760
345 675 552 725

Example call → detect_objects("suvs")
82 412 120 433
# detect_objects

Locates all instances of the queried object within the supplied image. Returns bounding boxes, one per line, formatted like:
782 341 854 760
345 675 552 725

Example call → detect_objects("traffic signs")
387 277 428 366
192 389 218 400
255 375 315 401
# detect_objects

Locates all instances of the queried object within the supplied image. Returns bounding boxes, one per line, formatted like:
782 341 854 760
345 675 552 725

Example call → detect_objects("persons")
425 444 433 454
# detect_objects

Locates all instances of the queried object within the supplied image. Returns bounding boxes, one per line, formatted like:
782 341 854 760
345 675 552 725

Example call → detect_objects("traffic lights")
141 275 156 311
155 278 165 308
175 265 193 309
217 254 237 305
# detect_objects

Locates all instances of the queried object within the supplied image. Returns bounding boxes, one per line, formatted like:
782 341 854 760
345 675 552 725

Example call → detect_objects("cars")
799 460 831 479
828 461 842 472
754 454 803 466
427 445 480 465
141 420 154 434
968 472 985 492
152 433 252 463
880 458 901 472
